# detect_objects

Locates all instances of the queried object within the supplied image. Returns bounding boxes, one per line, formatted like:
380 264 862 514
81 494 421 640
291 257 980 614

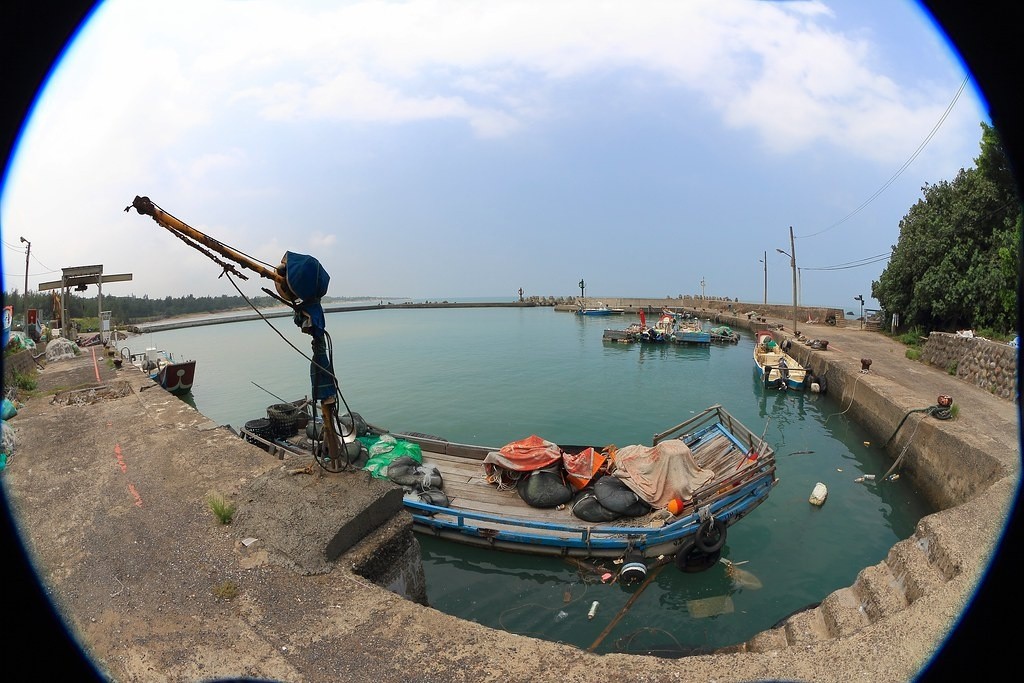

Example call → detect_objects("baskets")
267 404 299 438
245 419 276 452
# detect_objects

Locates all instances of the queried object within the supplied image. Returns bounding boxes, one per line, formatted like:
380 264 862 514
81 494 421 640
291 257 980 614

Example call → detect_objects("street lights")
760 258 769 305
776 248 797 335
854 294 863 330
20 236 31 336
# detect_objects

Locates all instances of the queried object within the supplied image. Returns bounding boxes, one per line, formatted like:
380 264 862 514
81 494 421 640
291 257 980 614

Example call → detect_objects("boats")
129 352 196 396
575 309 610 316
602 306 740 347
754 331 810 391
236 396 780 596
